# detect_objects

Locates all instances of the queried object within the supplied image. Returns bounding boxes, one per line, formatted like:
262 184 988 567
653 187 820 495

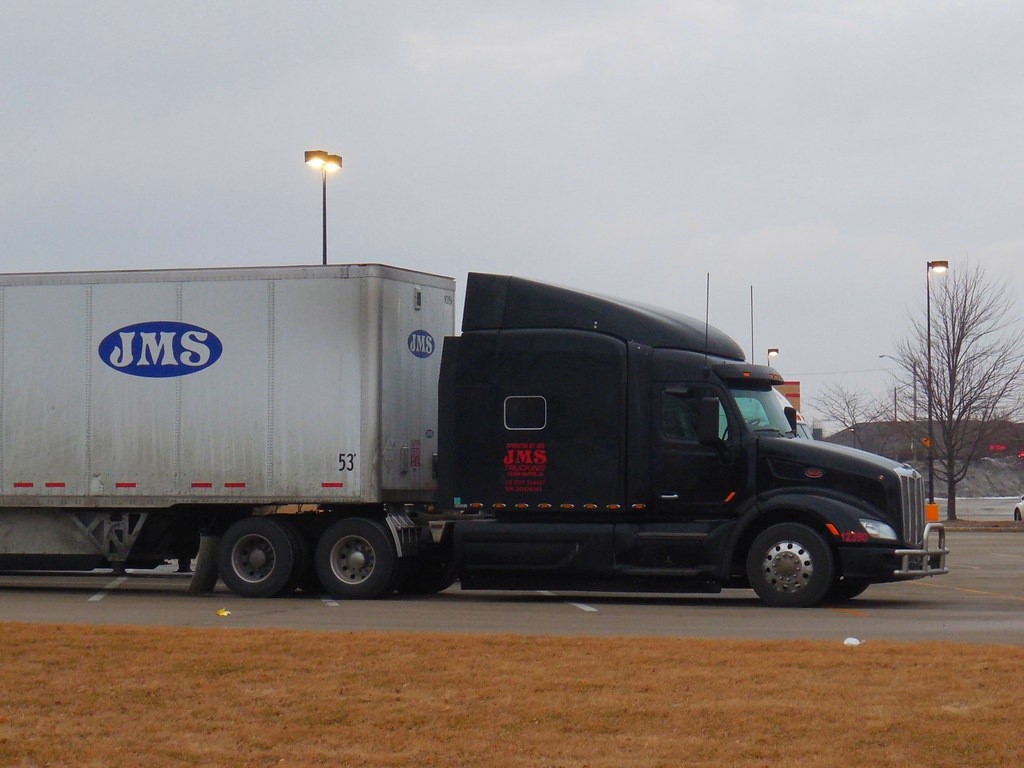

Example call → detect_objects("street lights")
924 260 949 505
304 151 344 264
766 348 779 367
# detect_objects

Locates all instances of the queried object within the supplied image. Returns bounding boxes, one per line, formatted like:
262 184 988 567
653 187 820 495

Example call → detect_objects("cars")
1014 495 1024 522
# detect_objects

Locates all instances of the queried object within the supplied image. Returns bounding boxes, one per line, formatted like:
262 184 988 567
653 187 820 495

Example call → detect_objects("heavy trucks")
0 262 953 611
